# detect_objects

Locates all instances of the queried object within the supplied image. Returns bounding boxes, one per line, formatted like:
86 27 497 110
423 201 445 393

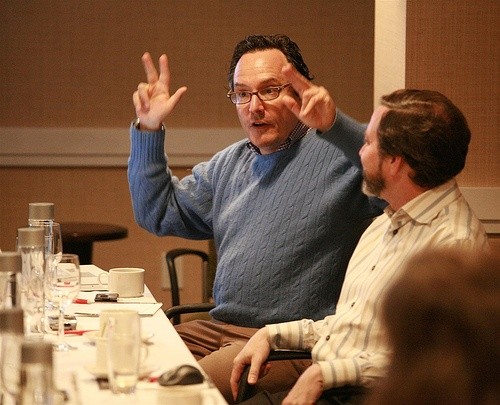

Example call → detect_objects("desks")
58 221 128 266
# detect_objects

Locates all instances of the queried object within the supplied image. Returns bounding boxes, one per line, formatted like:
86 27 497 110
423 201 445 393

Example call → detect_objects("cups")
97 310 140 393
98 268 145 298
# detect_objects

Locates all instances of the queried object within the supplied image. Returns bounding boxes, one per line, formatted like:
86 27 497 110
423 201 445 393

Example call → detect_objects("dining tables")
1 266 228 405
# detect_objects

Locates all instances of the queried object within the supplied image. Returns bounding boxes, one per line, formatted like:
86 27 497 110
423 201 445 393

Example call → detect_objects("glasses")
227 80 292 104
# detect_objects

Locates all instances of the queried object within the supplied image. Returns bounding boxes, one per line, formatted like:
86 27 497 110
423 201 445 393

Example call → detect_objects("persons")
126 33 388 405
362 243 500 405
230 87 489 405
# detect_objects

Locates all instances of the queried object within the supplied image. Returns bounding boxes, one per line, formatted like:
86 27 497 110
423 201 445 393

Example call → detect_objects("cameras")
48 314 77 331
95 293 119 302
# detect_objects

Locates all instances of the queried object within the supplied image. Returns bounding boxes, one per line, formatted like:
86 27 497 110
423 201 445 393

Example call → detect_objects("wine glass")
43 254 81 352
31 221 62 334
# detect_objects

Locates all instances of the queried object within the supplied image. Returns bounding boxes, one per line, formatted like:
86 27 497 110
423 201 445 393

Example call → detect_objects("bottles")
28 202 55 341
17 228 45 339
0 309 24 405
0 252 22 309
16 343 55 405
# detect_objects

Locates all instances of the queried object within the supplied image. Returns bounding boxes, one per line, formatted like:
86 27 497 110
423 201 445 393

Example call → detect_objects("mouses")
158 365 204 387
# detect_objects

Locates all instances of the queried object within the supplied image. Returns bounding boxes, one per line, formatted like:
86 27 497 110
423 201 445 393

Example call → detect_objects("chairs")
161 248 373 405
